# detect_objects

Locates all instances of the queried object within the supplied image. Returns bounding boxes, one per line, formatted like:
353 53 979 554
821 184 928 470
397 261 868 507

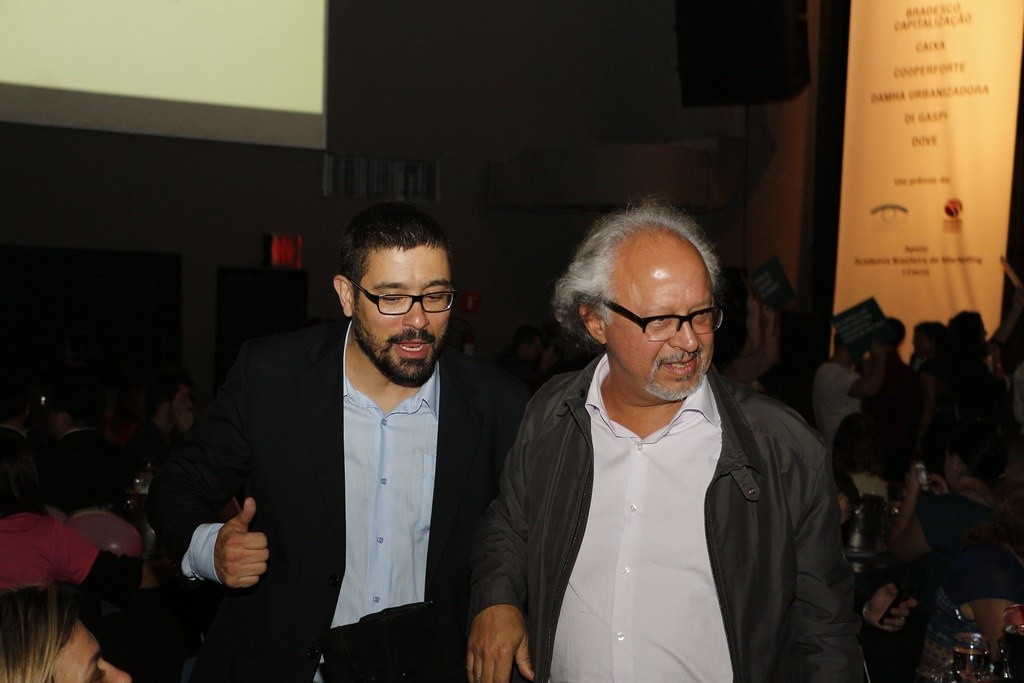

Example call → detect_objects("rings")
953 467 958 470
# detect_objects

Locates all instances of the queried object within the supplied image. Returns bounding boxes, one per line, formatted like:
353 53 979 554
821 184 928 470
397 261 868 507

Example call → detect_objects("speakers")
673 0 812 107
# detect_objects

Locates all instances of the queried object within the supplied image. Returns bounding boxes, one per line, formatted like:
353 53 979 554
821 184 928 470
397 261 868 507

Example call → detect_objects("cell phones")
879 588 915 625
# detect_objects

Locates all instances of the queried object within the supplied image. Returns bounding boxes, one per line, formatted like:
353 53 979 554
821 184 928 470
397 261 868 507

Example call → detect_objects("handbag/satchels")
318 599 449 683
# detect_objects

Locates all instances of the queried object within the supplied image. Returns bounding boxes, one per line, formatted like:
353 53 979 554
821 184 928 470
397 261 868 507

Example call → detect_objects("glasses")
344 280 457 315
603 298 724 342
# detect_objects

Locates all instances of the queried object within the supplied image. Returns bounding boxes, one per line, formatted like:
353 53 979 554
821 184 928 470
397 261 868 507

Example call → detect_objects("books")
743 255 798 316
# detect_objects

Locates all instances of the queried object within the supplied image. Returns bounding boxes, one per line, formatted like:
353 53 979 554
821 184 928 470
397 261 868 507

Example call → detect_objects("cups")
953 634 990 683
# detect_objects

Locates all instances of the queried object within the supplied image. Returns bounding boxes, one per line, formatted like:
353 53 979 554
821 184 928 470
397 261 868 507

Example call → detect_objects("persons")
0 435 172 596
814 283 1024 683
465 210 867 683
148 202 525 683
0 365 196 511
492 325 555 379
712 295 778 396
0 583 133 683
